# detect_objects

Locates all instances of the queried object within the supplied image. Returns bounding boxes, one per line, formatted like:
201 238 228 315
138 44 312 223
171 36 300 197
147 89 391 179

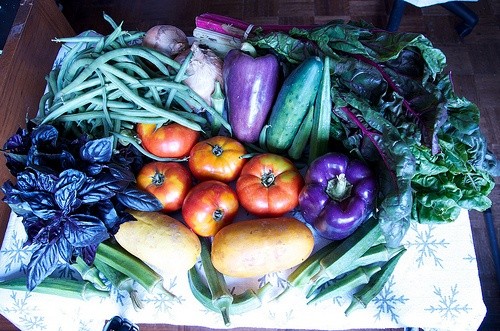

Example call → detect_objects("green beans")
35 9 233 164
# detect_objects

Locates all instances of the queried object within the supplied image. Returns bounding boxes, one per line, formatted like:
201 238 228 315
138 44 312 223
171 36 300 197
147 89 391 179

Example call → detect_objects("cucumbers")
265 57 324 153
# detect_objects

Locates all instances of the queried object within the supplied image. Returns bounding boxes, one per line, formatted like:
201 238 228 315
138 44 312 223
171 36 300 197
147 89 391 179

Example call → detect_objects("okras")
1 241 177 304
286 217 407 316
288 56 332 165
187 240 273 327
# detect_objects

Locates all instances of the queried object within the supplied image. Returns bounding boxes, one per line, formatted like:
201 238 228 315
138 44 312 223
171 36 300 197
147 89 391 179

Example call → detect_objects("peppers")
298 152 375 242
222 41 278 144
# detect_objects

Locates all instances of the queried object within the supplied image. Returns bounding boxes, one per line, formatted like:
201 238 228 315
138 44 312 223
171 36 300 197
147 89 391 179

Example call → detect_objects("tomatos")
136 120 303 237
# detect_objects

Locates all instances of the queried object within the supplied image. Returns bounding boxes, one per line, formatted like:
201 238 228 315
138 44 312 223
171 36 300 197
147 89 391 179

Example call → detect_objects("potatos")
211 218 314 280
112 211 201 274
